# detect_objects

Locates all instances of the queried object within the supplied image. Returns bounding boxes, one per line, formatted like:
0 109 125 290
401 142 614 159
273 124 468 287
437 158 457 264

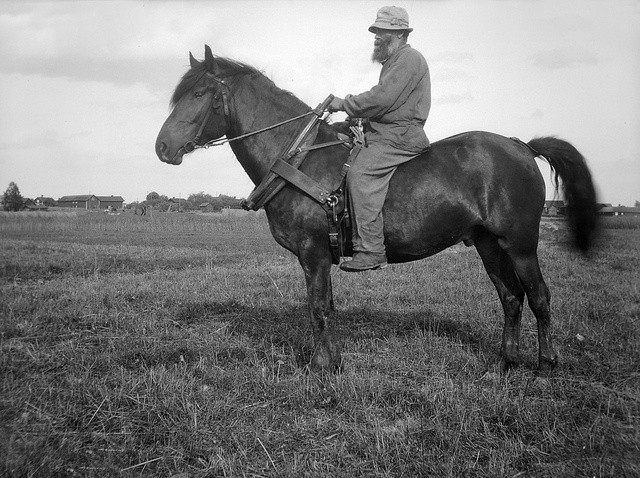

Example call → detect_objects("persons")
326 7 433 270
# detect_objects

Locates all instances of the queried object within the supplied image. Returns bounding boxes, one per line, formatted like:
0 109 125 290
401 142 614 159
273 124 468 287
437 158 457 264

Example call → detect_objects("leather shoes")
340 252 390 269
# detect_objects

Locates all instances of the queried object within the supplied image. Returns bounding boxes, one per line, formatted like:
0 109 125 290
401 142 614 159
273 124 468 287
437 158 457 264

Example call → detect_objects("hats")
369 7 412 33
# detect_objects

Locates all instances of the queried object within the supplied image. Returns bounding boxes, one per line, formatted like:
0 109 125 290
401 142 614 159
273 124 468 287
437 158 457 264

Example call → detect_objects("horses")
154 44 603 383
